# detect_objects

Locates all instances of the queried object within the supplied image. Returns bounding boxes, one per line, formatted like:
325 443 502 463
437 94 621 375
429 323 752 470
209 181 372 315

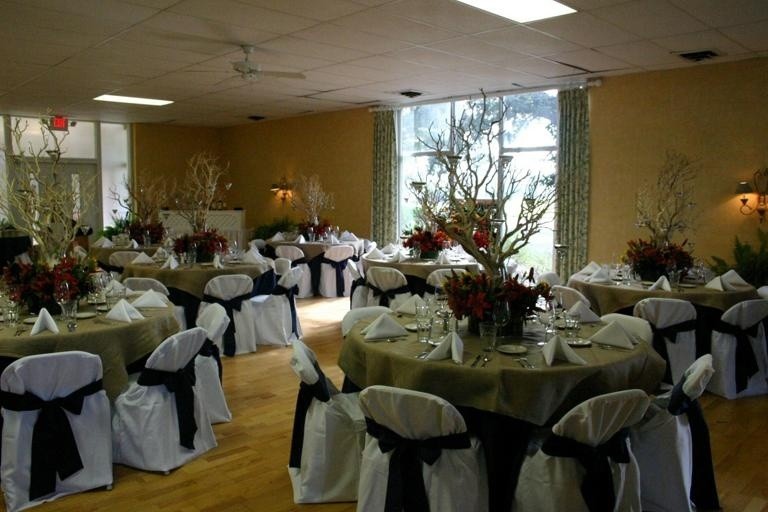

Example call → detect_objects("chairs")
538 262 768 401
2 272 230 510
348 240 518 306
288 300 713 510
249 229 373 299
74 230 300 359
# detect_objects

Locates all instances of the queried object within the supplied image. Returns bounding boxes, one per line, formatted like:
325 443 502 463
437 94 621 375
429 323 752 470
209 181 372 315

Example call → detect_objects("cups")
0 268 115 329
286 223 340 244
164 238 239 269
414 281 582 353
670 256 716 288
394 234 632 285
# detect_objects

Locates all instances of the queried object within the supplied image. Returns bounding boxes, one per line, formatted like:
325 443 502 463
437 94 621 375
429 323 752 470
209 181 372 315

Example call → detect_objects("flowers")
126 220 165 245
299 218 329 235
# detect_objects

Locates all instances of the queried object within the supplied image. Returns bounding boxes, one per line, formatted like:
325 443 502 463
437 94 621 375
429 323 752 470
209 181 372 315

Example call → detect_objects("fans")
183 43 307 87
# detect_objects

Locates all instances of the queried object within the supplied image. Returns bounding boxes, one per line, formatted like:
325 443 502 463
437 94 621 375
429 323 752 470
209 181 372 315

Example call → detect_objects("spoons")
481 354 492 368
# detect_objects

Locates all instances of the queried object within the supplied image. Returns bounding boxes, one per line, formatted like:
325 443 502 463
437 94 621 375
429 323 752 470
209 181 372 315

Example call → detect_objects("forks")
513 355 532 370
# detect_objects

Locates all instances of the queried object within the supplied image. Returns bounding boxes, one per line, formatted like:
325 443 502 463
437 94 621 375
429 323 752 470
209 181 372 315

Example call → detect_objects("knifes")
472 354 482 367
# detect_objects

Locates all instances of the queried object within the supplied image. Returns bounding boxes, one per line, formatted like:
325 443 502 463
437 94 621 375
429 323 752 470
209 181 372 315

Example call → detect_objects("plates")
404 321 420 331
562 336 592 347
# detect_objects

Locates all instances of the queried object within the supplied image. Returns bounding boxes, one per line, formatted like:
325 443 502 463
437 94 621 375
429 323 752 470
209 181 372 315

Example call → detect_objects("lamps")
269 174 289 206
732 169 767 224
